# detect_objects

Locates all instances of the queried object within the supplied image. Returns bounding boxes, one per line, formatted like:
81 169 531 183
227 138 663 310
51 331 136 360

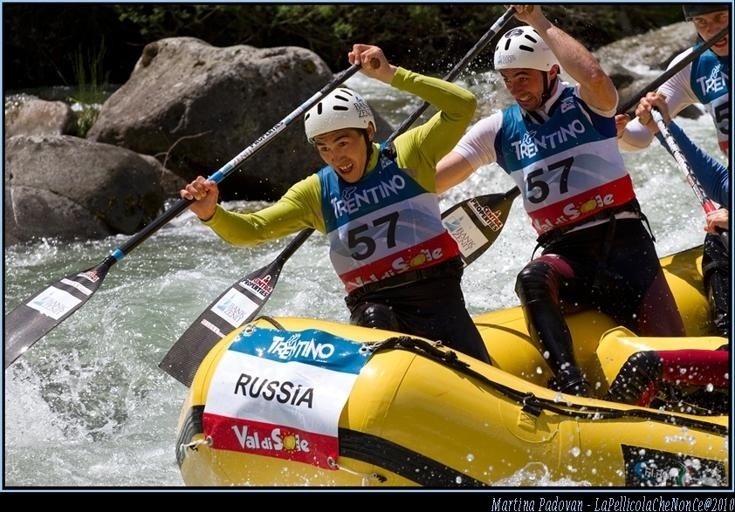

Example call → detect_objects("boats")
175 241 730 486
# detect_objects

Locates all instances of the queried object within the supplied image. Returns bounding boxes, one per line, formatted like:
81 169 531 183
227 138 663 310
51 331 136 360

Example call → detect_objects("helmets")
494 24 564 75
680 2 729 22
304 87 377 145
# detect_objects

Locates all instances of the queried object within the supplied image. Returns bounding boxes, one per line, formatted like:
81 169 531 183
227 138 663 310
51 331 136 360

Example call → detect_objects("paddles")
158 3 534 389
2 58 380 371
440 24 730 268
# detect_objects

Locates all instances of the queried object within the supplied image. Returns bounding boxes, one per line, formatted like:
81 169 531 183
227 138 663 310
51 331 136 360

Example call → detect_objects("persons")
179 44 494 367
434 5 711 428
615 3 731 241
635 90 732 351
